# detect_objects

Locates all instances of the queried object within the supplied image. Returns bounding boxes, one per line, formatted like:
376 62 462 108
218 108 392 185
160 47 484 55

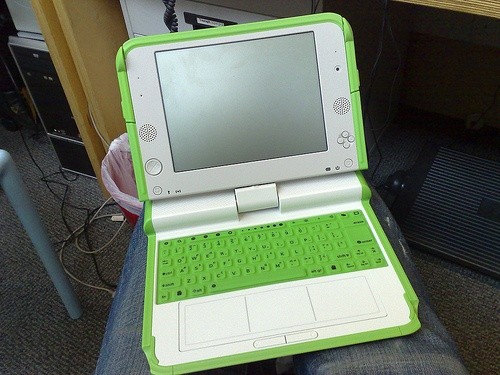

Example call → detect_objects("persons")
95 177 470 375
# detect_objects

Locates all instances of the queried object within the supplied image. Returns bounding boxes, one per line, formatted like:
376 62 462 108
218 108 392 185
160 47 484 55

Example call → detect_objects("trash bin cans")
100 132 144 228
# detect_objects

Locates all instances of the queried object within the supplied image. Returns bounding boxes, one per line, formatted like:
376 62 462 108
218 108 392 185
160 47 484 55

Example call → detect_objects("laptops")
117 11 422 375
